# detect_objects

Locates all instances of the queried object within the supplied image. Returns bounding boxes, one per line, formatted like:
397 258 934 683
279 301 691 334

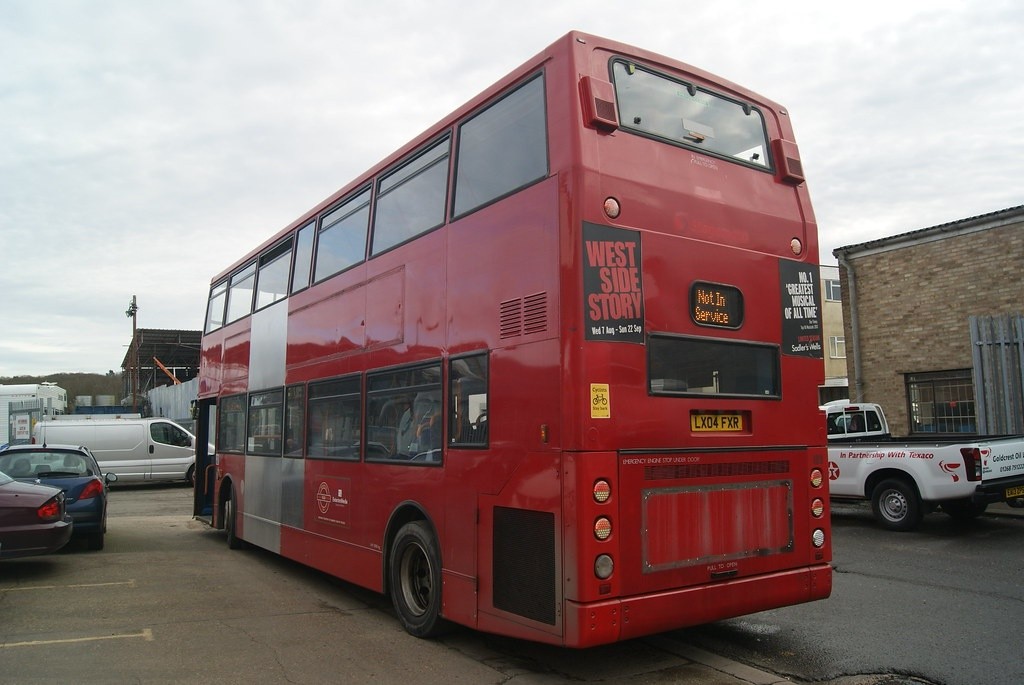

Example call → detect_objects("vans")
32 416 216 489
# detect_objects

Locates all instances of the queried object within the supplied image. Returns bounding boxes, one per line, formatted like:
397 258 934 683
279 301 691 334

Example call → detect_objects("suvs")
1 442 117 553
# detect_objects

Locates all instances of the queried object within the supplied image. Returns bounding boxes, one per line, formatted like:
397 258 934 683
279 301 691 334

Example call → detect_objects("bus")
193 27 836 652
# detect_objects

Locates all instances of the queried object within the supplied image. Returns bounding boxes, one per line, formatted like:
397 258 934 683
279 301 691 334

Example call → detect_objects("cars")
0 468 74 556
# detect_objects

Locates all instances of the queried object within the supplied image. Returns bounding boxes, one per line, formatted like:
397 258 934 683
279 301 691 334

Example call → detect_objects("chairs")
417 412 442 454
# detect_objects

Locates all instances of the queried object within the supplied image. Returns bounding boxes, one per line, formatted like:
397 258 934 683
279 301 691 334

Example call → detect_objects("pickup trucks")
817 403 1024 532
